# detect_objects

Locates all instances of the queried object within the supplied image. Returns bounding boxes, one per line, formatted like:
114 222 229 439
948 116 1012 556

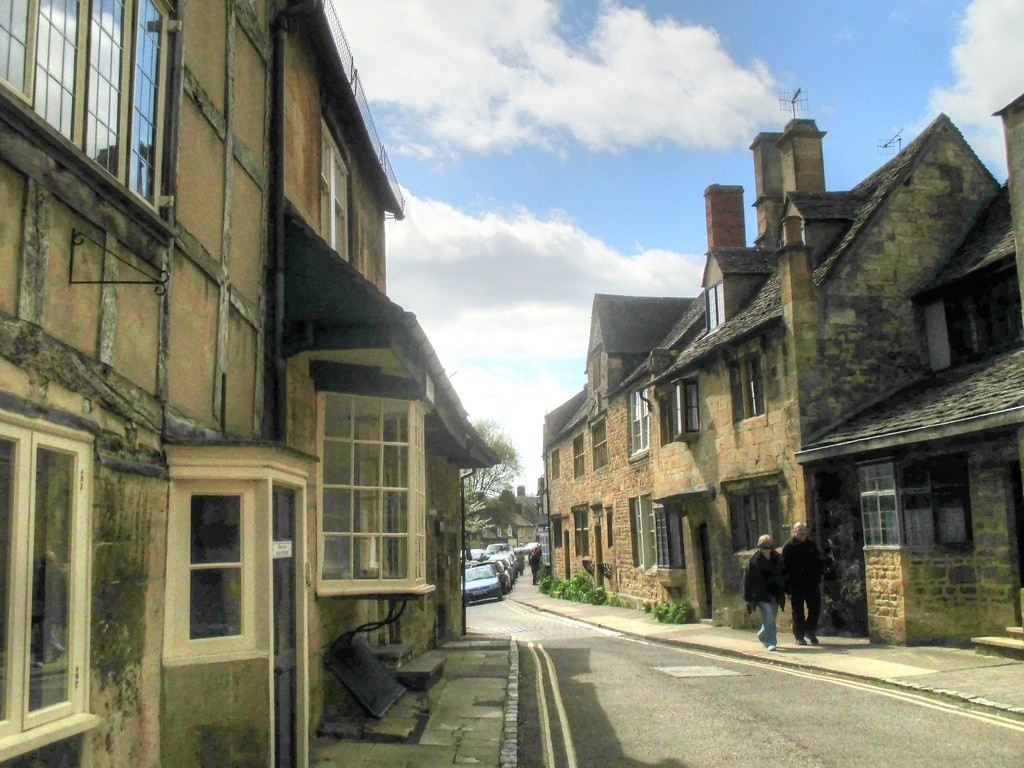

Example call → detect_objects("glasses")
761 545 773 550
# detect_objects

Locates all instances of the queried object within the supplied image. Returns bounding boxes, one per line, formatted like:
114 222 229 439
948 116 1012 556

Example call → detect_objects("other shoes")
757 631 769 647
795 638 808 645
768 645 777 651
805 631 819 646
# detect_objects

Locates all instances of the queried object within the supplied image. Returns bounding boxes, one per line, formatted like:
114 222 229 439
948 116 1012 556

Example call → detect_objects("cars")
461 564 504 606
459 541 538 594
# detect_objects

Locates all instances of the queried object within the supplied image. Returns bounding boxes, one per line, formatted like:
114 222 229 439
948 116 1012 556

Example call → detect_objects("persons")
519 551 525 575
746 535 784 651
530 544 542 586
787 519 829 645
